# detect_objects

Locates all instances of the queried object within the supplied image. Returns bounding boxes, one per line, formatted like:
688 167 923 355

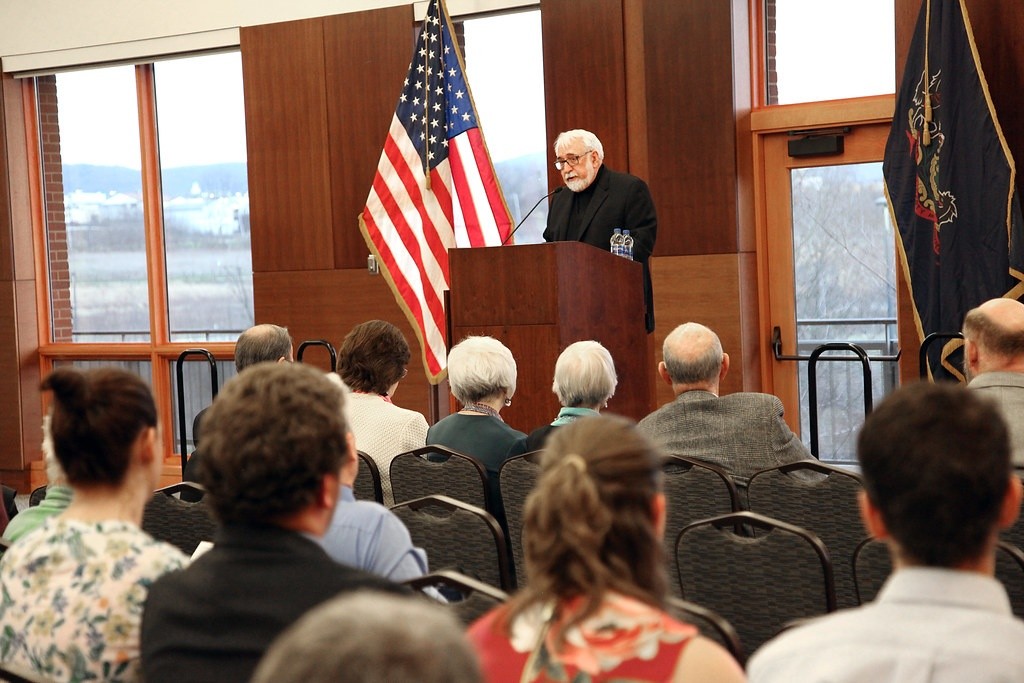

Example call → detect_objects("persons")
960 298 1024 485
506 340 619 459
741 381 1024 683
466 415 746 683
334 319 430 507
635 321 827 535
544 129 657 335
140 362 415 683
300 420 450 605
425 336 527 490
0 366 193 683
189 323 292 450
251 590 482 683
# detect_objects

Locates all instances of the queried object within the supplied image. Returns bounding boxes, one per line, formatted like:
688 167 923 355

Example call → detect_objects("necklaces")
461 402 504 421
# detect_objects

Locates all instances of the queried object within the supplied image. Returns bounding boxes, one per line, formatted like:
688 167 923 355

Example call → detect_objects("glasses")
555 149 594 171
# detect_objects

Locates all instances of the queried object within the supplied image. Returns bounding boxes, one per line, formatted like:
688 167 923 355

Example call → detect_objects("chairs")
141 443 1024 670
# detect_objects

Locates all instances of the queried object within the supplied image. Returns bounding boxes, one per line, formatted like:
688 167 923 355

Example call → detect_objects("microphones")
501 186 563 245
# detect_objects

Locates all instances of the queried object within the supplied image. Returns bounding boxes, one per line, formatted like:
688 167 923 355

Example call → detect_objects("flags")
358 0 514 386
881 0 1024 391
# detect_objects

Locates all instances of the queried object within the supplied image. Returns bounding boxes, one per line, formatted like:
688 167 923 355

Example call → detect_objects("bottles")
622 230 634 261
610 228 624 257
